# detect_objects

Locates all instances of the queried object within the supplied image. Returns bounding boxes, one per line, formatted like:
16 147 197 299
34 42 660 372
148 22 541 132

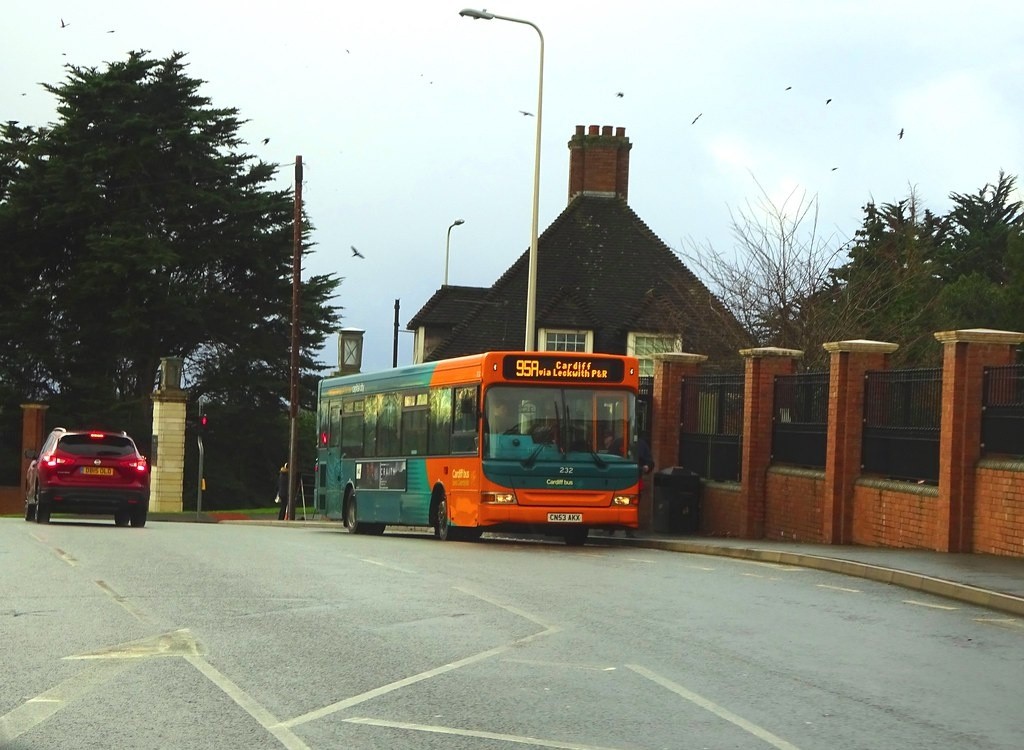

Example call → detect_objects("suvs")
24 426 150 528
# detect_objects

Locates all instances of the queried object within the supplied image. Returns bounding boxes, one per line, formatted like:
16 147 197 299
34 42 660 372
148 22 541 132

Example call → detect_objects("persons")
278 463 289 520
596 426 656 540
487 399 510 434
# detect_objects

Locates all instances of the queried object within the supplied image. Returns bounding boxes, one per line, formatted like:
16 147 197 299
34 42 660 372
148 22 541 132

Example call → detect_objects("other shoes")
624 534 635 538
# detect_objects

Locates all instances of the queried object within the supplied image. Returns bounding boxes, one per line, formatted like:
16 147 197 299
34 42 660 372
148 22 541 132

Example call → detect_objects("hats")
603 429 615 440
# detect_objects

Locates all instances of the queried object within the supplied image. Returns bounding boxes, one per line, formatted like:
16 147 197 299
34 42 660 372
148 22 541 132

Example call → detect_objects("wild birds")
20 18 115 96
261 49 702 260
784 86 905 173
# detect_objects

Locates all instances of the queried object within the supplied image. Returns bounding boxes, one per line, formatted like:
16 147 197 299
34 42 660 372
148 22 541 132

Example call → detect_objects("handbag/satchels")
275 492 281 504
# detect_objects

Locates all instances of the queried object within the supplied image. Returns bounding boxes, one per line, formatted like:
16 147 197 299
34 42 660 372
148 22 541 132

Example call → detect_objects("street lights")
457 7 545 437
444 219 466 285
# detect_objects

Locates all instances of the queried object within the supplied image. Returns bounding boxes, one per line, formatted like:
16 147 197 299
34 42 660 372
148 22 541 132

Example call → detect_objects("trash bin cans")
653 466 699 534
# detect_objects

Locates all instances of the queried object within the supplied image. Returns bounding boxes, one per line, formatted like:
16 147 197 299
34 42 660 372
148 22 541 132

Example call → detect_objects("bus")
313 351 641 546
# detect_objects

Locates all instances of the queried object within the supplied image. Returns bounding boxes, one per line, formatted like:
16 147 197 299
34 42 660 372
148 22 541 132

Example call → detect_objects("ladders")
296 473 314 521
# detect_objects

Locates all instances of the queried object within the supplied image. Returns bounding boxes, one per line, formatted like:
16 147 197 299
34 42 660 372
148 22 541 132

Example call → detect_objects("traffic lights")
198 416 208 436
